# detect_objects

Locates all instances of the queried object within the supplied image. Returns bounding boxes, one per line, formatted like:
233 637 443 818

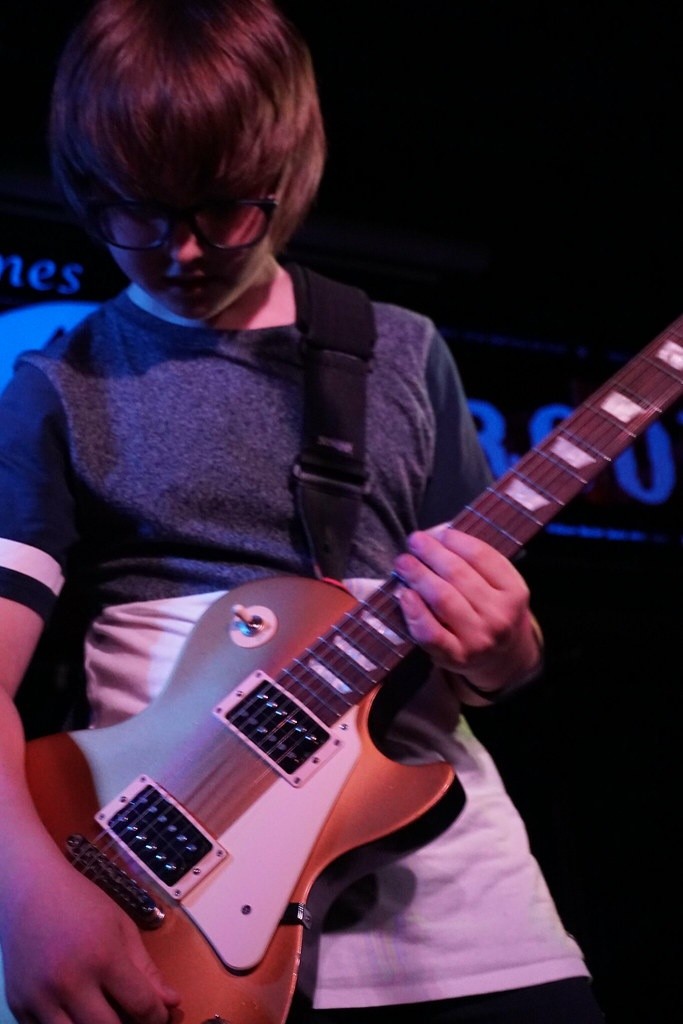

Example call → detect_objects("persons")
0 0 595 1024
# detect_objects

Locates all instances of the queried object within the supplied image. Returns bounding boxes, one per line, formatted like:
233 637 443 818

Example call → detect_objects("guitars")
0 317 683 1024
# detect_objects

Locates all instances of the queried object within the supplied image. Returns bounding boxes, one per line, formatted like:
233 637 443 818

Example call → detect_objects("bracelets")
463 616 547 701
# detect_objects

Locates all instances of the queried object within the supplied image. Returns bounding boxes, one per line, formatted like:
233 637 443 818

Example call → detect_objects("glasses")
88 160 293 252
